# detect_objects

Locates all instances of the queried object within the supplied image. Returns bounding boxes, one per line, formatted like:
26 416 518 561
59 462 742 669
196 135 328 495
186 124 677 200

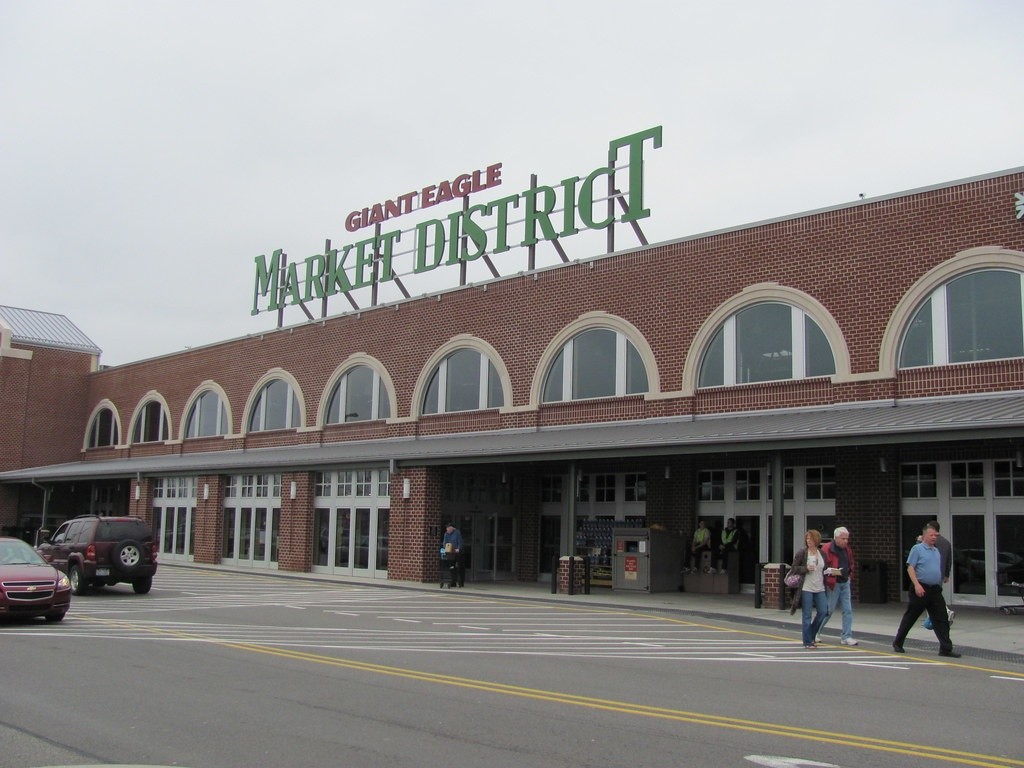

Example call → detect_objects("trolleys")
438 547 461 589
997 562 1024 615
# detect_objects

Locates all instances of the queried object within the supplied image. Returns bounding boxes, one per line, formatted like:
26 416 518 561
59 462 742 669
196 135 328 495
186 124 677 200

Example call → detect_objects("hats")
445 523 456 528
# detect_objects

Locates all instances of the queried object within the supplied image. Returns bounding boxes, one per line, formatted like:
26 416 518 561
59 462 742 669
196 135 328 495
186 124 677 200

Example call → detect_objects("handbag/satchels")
784 550 803 588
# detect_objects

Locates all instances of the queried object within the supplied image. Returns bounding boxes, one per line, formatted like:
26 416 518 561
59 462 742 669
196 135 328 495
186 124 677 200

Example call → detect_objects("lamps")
341 311 361 320
135 486 141 500
466 282 488 292
306 319 326 328
289 480 297 499
571 257 595 270
518 271 539 281
403 477 410 499
184 345 192 349
275 326 293 335
379 302 400 311
421 293 442 302
203 483 209 500
246 333 264 341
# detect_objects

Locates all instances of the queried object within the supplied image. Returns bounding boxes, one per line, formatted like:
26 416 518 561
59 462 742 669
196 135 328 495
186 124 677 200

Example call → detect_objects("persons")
893 520 961 659
708 518 740 576
791 529 835 649
679 520 711 573
815 527 858 645
443 524 465 586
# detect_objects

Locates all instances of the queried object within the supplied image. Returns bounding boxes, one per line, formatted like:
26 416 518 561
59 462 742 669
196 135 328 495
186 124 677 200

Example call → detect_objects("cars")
320 524 388 567
958 545 1020 574
0 537 72 627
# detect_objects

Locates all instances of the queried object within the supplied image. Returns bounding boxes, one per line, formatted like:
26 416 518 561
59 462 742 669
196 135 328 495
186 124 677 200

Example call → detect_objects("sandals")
803 644 818 649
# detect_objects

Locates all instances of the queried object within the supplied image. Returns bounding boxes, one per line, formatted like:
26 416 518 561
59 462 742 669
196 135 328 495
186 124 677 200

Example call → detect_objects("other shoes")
691 567 697 575
707 567 717 574
680 567 690 573
940 651 961 657
719 569 727 575
893 640 904 652
947 610 956 626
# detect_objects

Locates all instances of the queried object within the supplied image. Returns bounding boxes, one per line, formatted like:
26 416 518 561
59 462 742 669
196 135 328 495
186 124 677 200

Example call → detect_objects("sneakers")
815 638 821 643
841 637 858 646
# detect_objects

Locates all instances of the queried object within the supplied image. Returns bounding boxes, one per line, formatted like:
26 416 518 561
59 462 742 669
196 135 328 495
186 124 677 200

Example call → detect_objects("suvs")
37 514 158 595
158 521 196 555
